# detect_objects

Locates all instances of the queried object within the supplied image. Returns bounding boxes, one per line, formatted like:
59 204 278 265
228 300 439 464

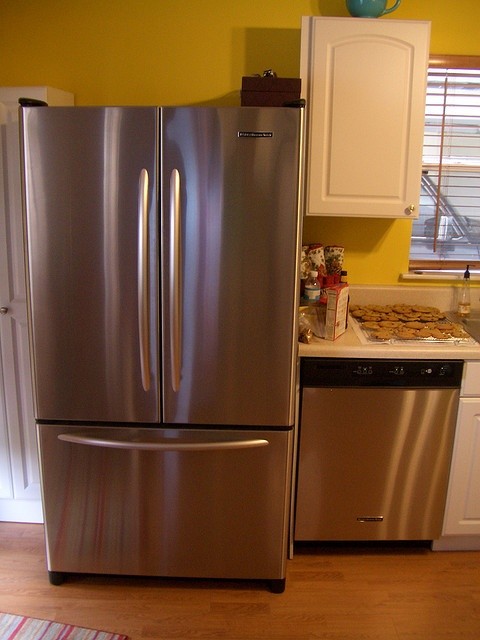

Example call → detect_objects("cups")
345 0 401 19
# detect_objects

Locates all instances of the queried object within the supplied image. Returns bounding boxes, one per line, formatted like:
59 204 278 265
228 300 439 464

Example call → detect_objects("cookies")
350 304 472 340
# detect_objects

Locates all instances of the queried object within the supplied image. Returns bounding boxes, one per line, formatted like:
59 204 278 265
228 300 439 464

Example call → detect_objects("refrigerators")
19 100 304 593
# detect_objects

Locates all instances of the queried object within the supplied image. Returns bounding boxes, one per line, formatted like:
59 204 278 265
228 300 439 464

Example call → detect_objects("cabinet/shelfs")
441 361 480 540
0 88 74 523
298 16 432 218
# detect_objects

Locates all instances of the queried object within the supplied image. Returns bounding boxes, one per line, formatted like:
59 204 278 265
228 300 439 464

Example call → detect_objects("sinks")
463 317 479 342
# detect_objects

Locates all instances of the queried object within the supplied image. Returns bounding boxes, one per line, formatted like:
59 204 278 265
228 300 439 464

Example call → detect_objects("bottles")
305 270 320 307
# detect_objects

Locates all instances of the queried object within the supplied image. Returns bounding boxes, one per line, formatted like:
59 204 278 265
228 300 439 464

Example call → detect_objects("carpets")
0 611 130 640
